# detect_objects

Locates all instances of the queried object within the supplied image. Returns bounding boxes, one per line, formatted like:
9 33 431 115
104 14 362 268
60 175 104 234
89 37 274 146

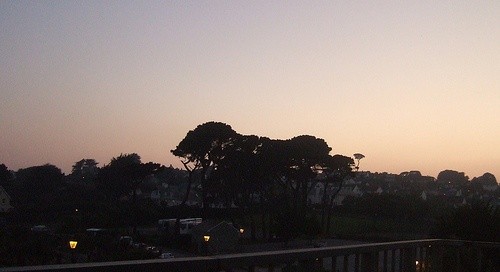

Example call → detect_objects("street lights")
70 240 77 264
204 236 210 256
239 229 245 253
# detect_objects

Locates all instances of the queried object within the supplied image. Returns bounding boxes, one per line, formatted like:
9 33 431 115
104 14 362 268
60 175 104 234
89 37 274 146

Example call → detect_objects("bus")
159 218 202 234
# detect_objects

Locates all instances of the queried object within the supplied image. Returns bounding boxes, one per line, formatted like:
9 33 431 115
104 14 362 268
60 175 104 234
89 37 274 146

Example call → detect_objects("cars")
31 225 47 232
87 227 174 259
